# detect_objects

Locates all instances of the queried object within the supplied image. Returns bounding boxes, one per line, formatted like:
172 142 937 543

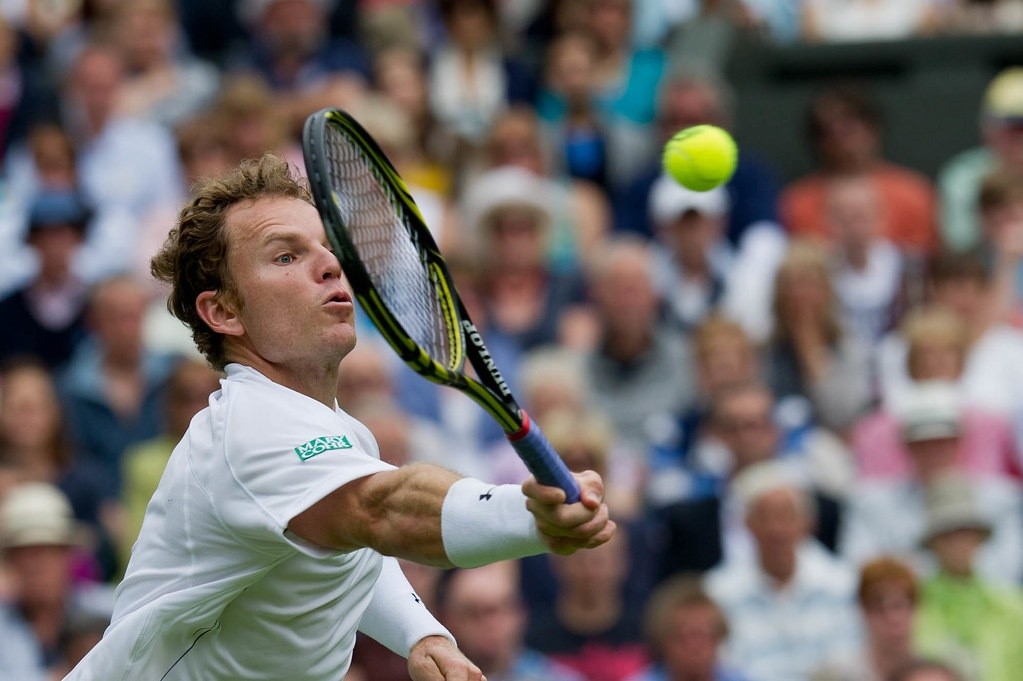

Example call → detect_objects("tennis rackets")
298 105 583 503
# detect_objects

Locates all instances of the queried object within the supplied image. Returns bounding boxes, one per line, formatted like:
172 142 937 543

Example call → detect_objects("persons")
0 0 1023 681
53 156 618 681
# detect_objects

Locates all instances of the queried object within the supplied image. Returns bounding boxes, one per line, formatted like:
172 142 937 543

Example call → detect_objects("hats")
648 175 724 223
914 472 995 551
979 67 1023 122
23 193 95 244
0 483 73 551
899 381 963 446
463 165 556 235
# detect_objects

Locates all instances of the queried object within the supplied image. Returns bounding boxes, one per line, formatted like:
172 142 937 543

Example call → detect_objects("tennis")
662 124 740 194
